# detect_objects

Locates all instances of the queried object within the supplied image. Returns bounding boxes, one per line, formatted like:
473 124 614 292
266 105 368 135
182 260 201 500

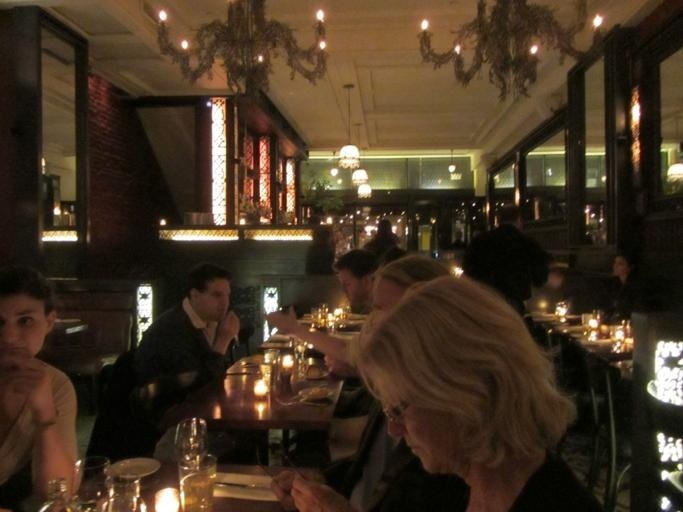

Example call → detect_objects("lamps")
449 149 455 172
417 0 603 104
357 184 372 198
157 0 329 105
339 83 363 158
338 158 359 169
668 113 683 184
352 169 369 184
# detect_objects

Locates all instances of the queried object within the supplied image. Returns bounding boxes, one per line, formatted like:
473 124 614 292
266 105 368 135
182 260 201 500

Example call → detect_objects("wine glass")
174 417 209 462
73 456 113 512
260 349 280 418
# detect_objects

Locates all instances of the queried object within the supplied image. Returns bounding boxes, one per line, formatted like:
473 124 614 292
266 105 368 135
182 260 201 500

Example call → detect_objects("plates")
111 457 161 478
298 388 332 400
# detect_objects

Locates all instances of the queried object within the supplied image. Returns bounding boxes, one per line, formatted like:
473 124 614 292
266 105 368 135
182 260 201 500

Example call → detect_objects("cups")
178 455 215 512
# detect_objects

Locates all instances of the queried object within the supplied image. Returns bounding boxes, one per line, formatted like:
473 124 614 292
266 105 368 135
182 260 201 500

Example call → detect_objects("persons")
336 214 355 255
290 273 606 512
264 246 410 420
134 261 257 465
331 249 377 303
304 214 336 274
0 264 81 511
468 202 564 317
366 218 399 262
269 254 453 511
452 230 467 251
566 251 653 392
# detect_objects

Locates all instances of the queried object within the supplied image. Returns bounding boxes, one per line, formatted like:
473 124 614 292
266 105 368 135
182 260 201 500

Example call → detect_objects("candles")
330 316 335 325
590 320 597 328
616 332 623 342
254 381 266 398
284 356 294 370
156 489 178 511
334 309 342 318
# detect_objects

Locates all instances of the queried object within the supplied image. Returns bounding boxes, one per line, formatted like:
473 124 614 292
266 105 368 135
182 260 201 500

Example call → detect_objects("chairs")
584 356 632 511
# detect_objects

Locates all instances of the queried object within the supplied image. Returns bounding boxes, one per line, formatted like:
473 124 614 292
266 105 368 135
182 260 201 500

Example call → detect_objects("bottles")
107 478 146 512
38 478 80 512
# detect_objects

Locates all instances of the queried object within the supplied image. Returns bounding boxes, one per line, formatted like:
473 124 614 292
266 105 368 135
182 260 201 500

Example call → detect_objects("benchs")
40 278 136 414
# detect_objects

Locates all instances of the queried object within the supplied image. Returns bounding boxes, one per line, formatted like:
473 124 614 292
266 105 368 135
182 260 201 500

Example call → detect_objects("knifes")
216 481 271 493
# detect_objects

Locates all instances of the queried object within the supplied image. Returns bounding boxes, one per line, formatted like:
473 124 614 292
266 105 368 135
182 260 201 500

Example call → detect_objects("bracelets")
32 407 66 429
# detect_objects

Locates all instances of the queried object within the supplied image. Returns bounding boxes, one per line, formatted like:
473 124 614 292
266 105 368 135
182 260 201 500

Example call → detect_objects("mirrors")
14 7 90 258
657 46 682 199
